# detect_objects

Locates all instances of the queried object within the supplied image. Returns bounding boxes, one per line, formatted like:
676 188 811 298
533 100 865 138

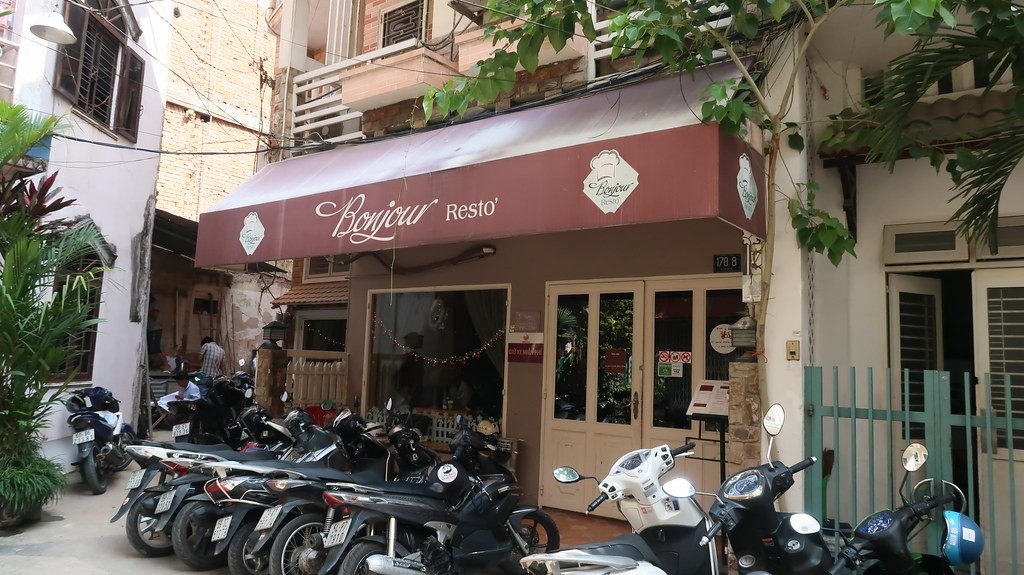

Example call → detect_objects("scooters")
521 439 719 575
662 403 834 575
791 443 956 575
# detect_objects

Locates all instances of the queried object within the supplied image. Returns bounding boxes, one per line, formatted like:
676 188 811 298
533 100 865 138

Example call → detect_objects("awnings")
194 54 767 268
816 81 1024 169
270 281 350 305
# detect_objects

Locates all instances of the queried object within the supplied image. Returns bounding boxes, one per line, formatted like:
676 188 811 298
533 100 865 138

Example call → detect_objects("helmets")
941 510 985 565
476 420 499 436
66 393 86 413
364 421 384 437
231 371 250 389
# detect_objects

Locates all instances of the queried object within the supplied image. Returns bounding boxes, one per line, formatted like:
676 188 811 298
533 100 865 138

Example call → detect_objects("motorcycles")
66 386 138 493
110 359 561 575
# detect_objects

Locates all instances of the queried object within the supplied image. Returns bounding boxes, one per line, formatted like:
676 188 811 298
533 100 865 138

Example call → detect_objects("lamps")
29 1 77 46
730 314 757 362
265 320 287 342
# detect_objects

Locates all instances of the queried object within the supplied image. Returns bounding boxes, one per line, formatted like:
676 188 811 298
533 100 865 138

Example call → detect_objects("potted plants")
0 96 108 529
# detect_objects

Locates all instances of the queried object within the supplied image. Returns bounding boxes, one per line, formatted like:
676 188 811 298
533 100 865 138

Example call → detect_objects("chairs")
141 382 172 430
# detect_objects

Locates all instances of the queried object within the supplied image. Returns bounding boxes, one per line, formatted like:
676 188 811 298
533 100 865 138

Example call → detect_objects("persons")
197 336 225 381
175 370 200 401
